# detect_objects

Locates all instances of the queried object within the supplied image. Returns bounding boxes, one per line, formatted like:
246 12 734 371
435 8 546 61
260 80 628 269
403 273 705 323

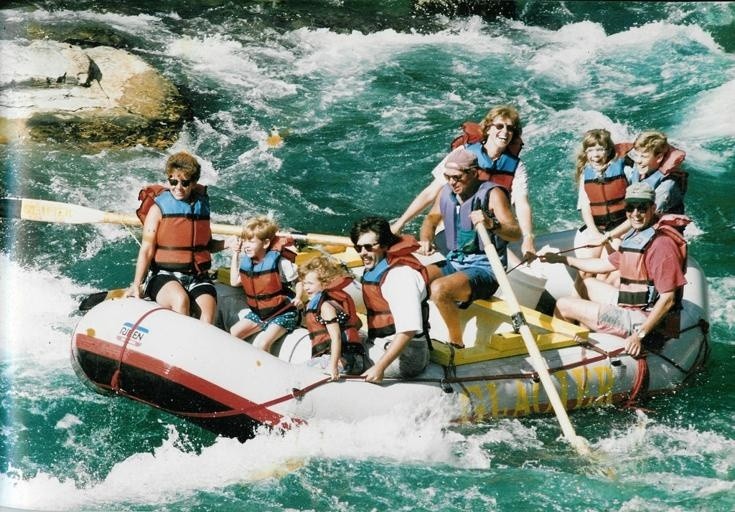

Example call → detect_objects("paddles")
0 197 353 247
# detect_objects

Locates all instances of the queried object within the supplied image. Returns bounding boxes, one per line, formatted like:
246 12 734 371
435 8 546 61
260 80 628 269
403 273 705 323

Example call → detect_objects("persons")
299 256 363 383
126 152 217 326
351 215 431 383
575 129 633 283
420 150 522 349
540 182 687 357
585 131 685 286
389 105 537 263
229 216 309 352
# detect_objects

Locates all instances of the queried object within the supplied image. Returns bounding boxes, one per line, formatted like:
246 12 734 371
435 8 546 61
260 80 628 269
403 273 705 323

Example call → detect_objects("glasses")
488 123 515 132
352 241 382 253
623 204 650 214
168 178 195 187
443 172 472 182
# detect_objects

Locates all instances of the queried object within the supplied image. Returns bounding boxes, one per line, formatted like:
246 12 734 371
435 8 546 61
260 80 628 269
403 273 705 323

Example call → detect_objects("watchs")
489 216 501 234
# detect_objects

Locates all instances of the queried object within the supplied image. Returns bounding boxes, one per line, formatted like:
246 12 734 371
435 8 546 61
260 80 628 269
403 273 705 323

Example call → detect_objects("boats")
68 256 709 438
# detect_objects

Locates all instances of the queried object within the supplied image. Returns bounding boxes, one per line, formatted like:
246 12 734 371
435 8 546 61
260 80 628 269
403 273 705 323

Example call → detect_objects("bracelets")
635 325 648 339
520 231 535 241
605 232 613 241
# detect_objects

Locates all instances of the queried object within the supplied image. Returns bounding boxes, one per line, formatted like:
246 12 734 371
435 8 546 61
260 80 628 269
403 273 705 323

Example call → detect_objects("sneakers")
449 343 466 349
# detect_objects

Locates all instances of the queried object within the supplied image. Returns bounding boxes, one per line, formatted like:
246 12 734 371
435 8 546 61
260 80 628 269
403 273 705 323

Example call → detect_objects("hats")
444 148 485 171
623 182 656 203
